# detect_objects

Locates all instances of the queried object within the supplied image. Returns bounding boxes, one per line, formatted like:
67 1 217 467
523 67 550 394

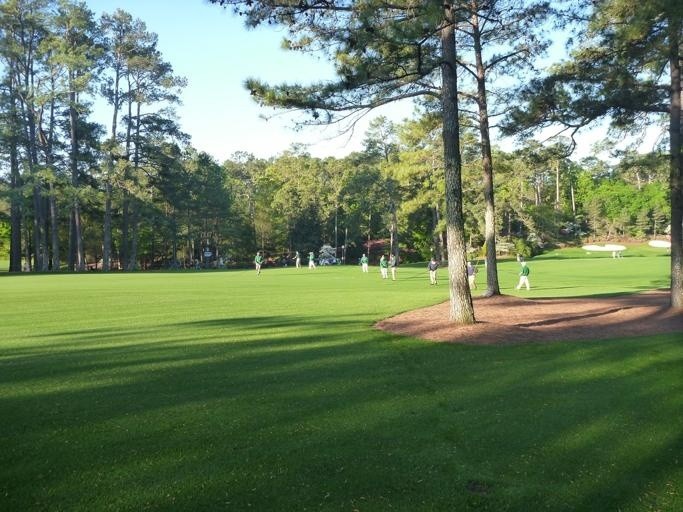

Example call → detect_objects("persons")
360 253 369 272
427 257 439 284
612 250 623 259
390 254 397 280
19 255 235 273
516 253 524 263
307 251 316 269
515 262 530 291
291 250 302 269
254 251 264 275
380 254 388 278
467 261 477 290
